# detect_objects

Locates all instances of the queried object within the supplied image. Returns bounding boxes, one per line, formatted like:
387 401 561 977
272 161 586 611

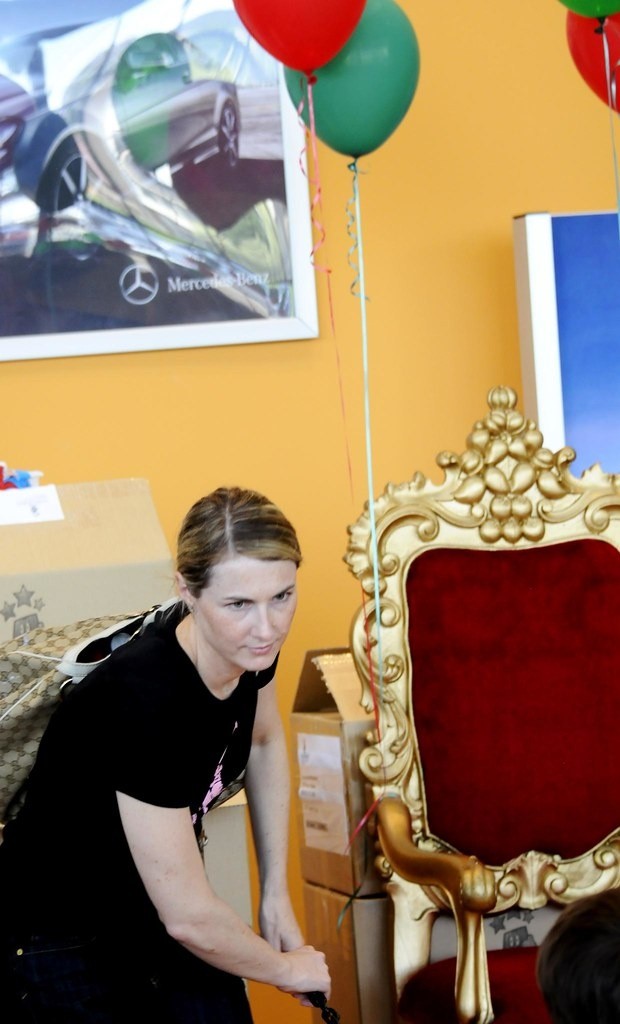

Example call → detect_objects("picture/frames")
0 1 322 360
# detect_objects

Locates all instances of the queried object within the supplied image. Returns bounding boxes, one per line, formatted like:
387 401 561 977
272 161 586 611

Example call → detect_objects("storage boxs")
0 477 175 648
291 648 566 1023
202 793 254 925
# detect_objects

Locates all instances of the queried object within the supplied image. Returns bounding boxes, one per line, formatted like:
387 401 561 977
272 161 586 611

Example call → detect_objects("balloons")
233 0 367 81
558 0 620 21
284 0 421 171
565 14 620 110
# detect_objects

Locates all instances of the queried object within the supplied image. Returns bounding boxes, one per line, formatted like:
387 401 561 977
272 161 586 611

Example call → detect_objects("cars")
1 18 244 266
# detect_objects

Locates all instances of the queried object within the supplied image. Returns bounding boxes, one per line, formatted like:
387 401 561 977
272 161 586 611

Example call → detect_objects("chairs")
341 385 620 1023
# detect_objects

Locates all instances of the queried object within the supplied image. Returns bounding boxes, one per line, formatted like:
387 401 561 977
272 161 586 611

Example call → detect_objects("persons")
2 483 334 1024
535 889 620 1023
